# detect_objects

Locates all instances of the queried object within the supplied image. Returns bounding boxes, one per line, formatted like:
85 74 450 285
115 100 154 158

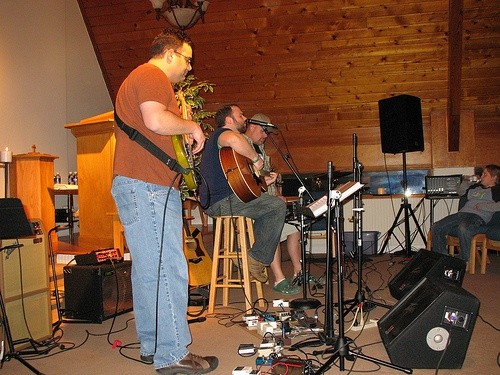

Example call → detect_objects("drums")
276 196 304 223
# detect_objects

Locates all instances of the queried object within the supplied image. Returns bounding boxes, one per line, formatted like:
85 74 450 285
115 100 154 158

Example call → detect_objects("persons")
198 103 287 282
110 28 218 375
239 113 327 295
431 165 500 262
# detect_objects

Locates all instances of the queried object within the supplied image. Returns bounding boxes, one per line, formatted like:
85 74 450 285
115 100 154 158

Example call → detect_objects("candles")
0 146 13 162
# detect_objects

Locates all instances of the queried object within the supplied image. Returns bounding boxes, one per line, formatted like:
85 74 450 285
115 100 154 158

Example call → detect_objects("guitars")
170 83 198 191
181 201 213 286
217 142 269 204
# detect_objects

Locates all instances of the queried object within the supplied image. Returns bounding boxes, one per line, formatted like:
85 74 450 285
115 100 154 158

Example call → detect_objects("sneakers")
156 352 219 375
248 258 268 283
232 259 243 269
140 355 154 362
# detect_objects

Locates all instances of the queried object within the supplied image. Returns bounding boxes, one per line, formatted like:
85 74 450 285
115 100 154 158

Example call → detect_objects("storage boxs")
344 231 380 255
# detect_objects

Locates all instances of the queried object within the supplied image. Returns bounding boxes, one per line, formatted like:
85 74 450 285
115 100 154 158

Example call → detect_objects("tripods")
290 134 413 375
379 153 426 255
0 198 101 375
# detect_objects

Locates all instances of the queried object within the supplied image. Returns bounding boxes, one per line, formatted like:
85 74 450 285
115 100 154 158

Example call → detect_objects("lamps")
148 1 210 31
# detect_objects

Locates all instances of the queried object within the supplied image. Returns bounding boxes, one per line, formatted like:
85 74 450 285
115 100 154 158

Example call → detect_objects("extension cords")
242 310 289 356
351 322 377 332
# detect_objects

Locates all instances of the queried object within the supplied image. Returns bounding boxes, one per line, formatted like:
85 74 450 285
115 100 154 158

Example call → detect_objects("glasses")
165 49 191 63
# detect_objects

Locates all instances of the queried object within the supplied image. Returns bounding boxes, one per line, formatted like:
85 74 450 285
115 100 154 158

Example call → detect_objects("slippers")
292 275 323 285
272 280 301 295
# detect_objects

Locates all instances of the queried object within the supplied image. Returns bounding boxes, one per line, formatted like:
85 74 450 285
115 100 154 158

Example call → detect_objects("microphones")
246 119 277 129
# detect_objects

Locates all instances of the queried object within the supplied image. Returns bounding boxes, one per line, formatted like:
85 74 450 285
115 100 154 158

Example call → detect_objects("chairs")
304 192 336 259
427 229 500 276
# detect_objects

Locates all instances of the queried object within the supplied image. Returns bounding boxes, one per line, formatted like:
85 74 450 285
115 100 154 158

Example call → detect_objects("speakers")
64 260 131 323
379 95 424 155
0 218 49 302
5 290 52 353
377 248 480 370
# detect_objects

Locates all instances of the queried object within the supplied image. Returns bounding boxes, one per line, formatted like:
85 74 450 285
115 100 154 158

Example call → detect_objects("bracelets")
252 155 261 163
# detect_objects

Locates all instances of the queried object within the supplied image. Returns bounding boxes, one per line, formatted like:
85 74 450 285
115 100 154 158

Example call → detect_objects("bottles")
54 172 62 184
68 172 78 185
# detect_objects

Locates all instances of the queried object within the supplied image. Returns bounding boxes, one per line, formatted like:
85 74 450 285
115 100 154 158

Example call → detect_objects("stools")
206 216 265 314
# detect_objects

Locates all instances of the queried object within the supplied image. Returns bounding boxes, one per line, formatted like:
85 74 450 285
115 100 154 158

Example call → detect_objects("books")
305 180 360 218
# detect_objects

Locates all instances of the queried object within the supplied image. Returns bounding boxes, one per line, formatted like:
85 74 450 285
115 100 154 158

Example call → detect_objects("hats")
251 114 277 134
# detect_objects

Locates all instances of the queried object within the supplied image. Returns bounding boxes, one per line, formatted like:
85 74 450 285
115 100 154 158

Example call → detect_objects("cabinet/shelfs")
10 111 129 265
